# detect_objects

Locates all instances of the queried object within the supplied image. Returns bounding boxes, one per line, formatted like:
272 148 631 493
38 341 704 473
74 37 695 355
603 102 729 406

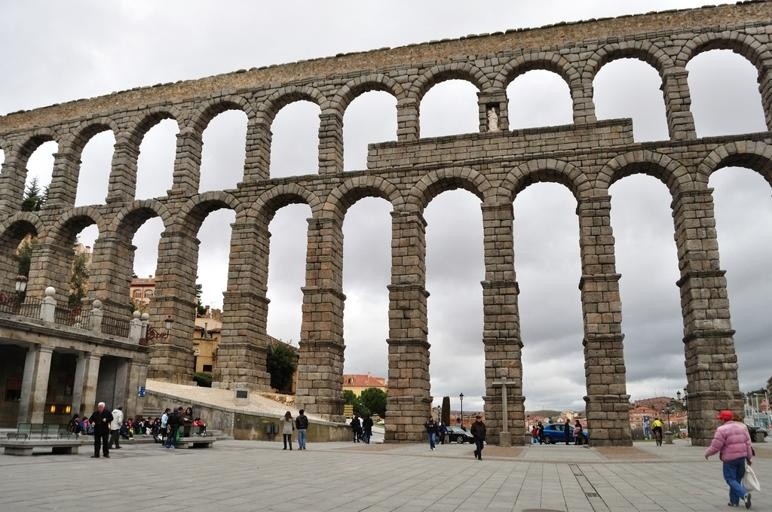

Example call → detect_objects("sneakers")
727 502 739 507
478 459 483 460
283 447 305 451
743 492 751 509
90 444 122 458
474 450 477 458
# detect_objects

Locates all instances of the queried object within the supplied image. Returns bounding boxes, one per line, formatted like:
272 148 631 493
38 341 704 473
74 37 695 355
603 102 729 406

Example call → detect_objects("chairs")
5 419 84 440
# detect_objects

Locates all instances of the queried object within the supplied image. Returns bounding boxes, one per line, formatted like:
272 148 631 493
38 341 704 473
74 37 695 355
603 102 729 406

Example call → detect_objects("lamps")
145 315 176 344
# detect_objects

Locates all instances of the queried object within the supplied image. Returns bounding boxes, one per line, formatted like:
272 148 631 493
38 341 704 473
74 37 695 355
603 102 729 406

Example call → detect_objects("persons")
67 406 206 448
362 415 373 444
350 416 360 443
705 410 752 509
471 416 486 460
89 403 114 458
531 426 540 443
424 416 447 451
280 411 295 450
573 420 582 445
538 422 544 445
651 418 663 440
295 410 308 450
564 419 571 445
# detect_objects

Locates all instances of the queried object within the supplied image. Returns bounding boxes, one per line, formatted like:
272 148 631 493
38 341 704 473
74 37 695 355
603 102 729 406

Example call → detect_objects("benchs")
1 437 85 457
163 433 218 449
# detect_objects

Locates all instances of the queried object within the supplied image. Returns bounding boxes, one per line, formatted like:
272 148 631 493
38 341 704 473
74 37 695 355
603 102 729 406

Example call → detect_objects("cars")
744 424 769 443
442 425 475 446
537 422 589 445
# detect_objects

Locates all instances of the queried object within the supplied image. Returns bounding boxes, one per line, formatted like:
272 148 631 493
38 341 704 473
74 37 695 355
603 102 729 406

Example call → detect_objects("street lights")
661 404 677 432
459 393 465 427
526 413 530 433
677 388 690 436
